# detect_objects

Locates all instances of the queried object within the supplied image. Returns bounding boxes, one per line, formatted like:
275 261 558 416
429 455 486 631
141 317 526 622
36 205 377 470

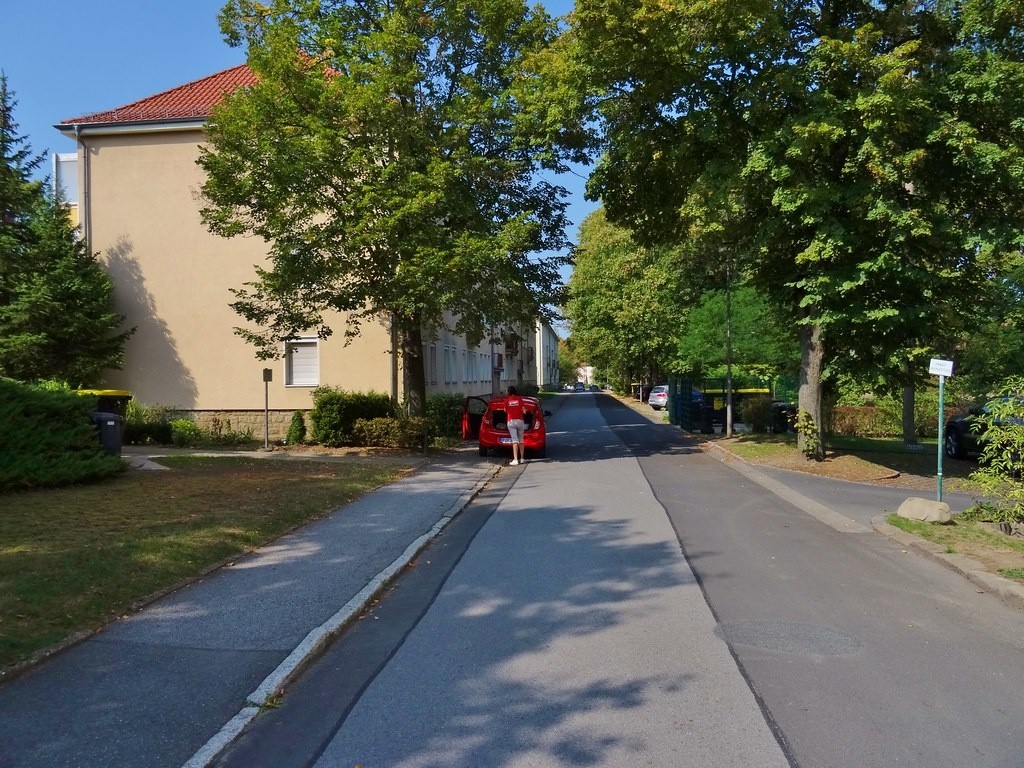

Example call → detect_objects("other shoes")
520 458 524 463
509 459 518 465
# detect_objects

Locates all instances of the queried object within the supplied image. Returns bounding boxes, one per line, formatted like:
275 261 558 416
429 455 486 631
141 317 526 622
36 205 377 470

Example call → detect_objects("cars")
574 383 584 392
636 386 653 403
590 385 598 390
649 385 703 411
462 395 551 459
944 395 1024 478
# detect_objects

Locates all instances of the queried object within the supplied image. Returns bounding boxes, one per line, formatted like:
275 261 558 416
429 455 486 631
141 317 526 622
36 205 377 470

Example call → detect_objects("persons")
504 386 526 466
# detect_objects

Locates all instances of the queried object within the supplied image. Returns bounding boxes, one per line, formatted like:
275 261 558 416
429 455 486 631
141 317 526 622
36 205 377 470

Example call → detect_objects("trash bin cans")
75 388 132 444
93 410 124 463
630 383 640 399
